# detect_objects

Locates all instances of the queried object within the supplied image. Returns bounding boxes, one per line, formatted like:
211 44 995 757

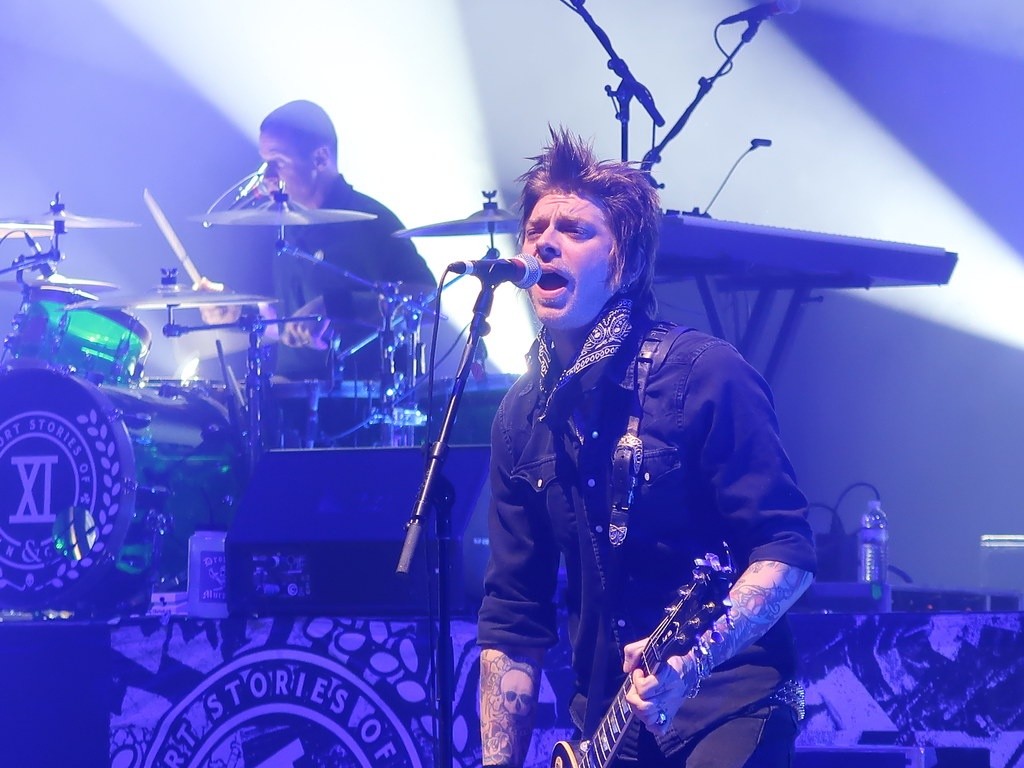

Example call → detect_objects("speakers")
221 443 494 620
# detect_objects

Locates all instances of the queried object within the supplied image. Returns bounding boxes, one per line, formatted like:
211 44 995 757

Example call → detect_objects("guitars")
550 542 737 768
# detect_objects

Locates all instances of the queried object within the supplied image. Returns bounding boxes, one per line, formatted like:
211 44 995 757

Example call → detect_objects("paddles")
0 211 142 239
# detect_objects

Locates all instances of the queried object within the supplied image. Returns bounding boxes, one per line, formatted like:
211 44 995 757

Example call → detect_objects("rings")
303 341 312 346
656 713 666 726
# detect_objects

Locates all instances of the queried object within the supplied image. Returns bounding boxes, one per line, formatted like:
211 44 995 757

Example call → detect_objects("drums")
418 372 520 445
11 290 152 389
1 360 178 615
271 380 383 447
143 377 246 447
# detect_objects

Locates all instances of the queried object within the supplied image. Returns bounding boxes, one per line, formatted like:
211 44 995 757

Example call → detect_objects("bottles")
861 500 888 582
128 413 152 447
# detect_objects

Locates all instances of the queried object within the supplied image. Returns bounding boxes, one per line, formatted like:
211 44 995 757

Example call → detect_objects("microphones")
721 0 800 24
325 329 346 371
25 233 57 279
448 253 542 289
205 182 272 231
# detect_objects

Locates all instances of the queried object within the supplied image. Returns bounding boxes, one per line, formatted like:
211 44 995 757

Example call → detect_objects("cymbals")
182 203 380 231
391 209 524 240
65 279 279 312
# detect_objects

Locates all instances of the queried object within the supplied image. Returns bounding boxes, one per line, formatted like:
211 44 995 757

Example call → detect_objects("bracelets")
689 649 703 700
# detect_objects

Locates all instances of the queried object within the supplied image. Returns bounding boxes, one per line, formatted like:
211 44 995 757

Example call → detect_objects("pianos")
654 210 960 290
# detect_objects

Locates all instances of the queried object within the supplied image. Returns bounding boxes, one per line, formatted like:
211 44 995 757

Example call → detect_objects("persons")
192 102 439 372
476 129 817 768
